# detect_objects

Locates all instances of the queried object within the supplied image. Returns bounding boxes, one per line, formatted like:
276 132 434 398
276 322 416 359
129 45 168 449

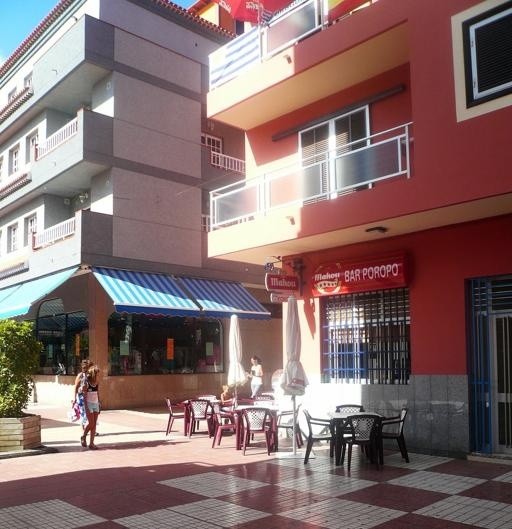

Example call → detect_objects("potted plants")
0 319 44 450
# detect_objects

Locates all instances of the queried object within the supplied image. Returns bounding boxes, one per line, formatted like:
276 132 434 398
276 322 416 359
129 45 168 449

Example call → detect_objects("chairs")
379 408 409 465
303 409 336 464
336 405 364 453
340 414 383 466
165 395 304 455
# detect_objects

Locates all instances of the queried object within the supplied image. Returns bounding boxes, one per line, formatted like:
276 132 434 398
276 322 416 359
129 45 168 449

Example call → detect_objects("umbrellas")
275 295 316 460
227 314 249 409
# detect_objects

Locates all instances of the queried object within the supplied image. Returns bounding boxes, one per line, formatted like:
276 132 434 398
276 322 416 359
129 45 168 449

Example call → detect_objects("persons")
245 355 264 400
71 359 99 436
81 368 100 450
220 384 236 434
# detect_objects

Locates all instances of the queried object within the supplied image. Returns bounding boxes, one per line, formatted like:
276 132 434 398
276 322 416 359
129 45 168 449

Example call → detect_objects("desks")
328 411 385 465
